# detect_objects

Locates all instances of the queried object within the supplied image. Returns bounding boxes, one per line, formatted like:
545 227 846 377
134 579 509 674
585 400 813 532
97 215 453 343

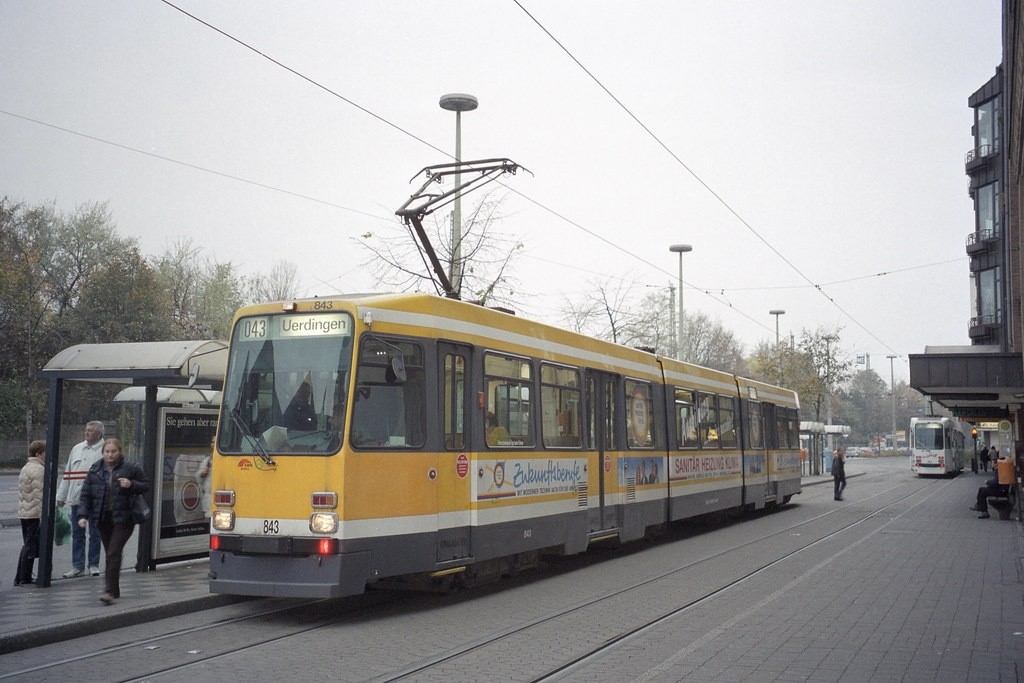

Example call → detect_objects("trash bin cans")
826 451 834 471
800 450 806 461
997 457 1019 485
971 458 976 472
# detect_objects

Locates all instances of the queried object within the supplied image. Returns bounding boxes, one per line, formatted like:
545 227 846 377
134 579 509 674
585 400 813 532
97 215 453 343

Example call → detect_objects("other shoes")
100 592 114 602
32 578 37 583
970 504 980 510
14 581 20 585
834 497 843 501
978 513 989 518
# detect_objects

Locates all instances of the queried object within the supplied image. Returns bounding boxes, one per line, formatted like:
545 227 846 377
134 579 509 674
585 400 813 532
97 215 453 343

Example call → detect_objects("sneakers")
63 568 83 577
89 566 99 576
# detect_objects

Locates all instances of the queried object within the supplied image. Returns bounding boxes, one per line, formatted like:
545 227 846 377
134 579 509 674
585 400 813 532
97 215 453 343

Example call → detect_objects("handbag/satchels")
28 526 40 558
130 462 151 523
55 505 73 546
980 463 982 470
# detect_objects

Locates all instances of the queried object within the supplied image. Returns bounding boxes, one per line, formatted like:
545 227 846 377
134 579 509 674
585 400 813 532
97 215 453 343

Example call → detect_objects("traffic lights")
972 428 977 439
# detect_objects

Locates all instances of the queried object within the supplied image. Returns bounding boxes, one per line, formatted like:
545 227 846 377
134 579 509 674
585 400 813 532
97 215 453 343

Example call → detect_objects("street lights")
887 355 898 456
440 93 480 295
823 336 834 452
670 245 693 360
768 310 785 386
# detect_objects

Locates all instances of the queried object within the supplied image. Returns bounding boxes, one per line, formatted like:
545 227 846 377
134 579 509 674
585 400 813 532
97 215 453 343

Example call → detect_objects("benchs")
987 496 1014 520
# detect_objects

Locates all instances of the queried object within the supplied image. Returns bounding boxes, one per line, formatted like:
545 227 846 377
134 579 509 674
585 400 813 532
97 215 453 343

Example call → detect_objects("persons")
284 382 318 450
13 440 47 585
969 458 1008 519
987 445 997 464
980 446 990 472
76 438 150 604
831 447 847 501
196 435 216 519
484 411 514 447
55 421 105 578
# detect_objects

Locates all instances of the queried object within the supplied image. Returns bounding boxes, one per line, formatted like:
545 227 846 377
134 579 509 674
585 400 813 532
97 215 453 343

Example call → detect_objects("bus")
185 293 802 597
909 417 965 477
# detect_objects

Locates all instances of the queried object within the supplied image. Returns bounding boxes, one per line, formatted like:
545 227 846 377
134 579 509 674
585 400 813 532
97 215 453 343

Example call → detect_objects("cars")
861 446 910 453
846 447 862 457
